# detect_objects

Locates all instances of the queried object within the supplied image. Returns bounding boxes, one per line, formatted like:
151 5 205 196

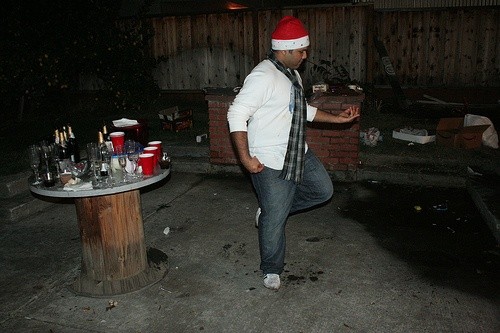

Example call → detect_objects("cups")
138 153 154 175
158 155 171 169
109 131 125 154
57 159 73 183
143 146 158 167
148 141 162 159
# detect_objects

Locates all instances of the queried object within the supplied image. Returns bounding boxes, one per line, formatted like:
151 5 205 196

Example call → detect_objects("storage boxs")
392 130 436 145
158 106 194 131
434 117 491 150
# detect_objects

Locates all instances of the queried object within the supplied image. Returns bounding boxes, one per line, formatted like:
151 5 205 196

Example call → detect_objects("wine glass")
27 139 144 186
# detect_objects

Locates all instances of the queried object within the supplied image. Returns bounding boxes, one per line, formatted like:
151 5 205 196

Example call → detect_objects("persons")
227 16 360 290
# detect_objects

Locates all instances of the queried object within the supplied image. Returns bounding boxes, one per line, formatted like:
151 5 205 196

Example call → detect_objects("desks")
28 164 171 299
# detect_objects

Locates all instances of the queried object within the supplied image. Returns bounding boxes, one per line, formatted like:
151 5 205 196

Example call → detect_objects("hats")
272 15 310 51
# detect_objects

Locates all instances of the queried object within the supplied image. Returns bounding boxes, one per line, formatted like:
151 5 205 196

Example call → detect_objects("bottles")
96 131 109 176
58 132 71 161
67 126 80 162
63 126 69 143
134 128 144 153
102 124 112 152
39 151 55 188
53 129 60 154
